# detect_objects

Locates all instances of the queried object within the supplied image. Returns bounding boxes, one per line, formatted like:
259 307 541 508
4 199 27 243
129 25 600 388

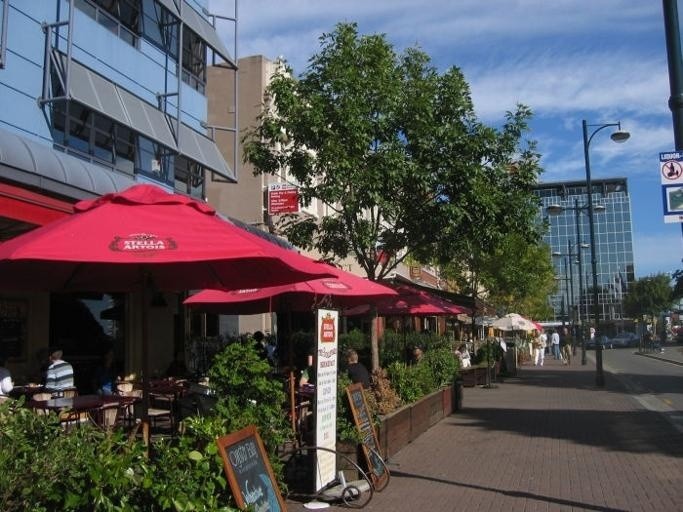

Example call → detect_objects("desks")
8 377 190 435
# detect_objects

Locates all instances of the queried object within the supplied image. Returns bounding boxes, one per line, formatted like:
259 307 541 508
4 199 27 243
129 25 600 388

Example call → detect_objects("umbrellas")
340 284 473 346
492 312 544 378
182 269 399 431
0 184 339 460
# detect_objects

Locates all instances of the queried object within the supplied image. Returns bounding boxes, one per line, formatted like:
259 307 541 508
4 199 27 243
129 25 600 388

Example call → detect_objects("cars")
584 331 661 350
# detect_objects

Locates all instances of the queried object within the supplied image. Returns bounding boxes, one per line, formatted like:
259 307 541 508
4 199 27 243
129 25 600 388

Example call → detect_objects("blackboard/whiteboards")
215 424 288 512
345 382 388 490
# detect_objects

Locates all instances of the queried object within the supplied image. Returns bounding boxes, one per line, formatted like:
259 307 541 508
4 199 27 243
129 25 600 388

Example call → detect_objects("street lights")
581 118 630 385
546 198 606 363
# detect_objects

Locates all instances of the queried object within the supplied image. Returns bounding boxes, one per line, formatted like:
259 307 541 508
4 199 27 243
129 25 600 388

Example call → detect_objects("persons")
411 344 424 364
0 353 15 398
454 342 472 369
44 347 74 389
530 327 575 365
299 363 313 388
345 349 370 389
